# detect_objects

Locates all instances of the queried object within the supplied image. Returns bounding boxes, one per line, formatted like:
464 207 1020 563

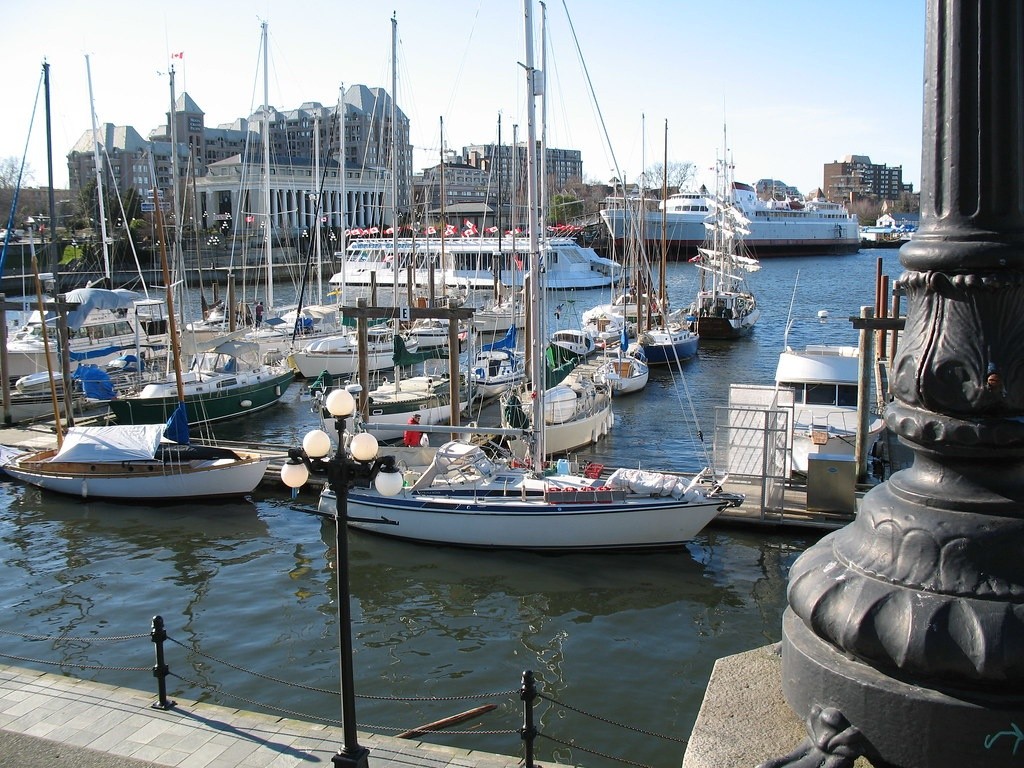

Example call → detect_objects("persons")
404 413 423 447
256 302 264 327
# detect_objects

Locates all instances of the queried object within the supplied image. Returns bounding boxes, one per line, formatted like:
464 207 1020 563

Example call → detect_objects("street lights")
280 393 379 767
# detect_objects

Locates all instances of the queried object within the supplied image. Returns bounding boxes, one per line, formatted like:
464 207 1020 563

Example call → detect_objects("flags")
245 216 254 223
321 217 582 238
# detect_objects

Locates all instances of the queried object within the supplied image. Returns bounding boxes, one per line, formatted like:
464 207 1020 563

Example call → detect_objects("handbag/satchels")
420 433 429 448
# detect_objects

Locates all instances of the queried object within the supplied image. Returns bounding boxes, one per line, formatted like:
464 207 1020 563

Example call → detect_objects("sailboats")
1 3 910 552
